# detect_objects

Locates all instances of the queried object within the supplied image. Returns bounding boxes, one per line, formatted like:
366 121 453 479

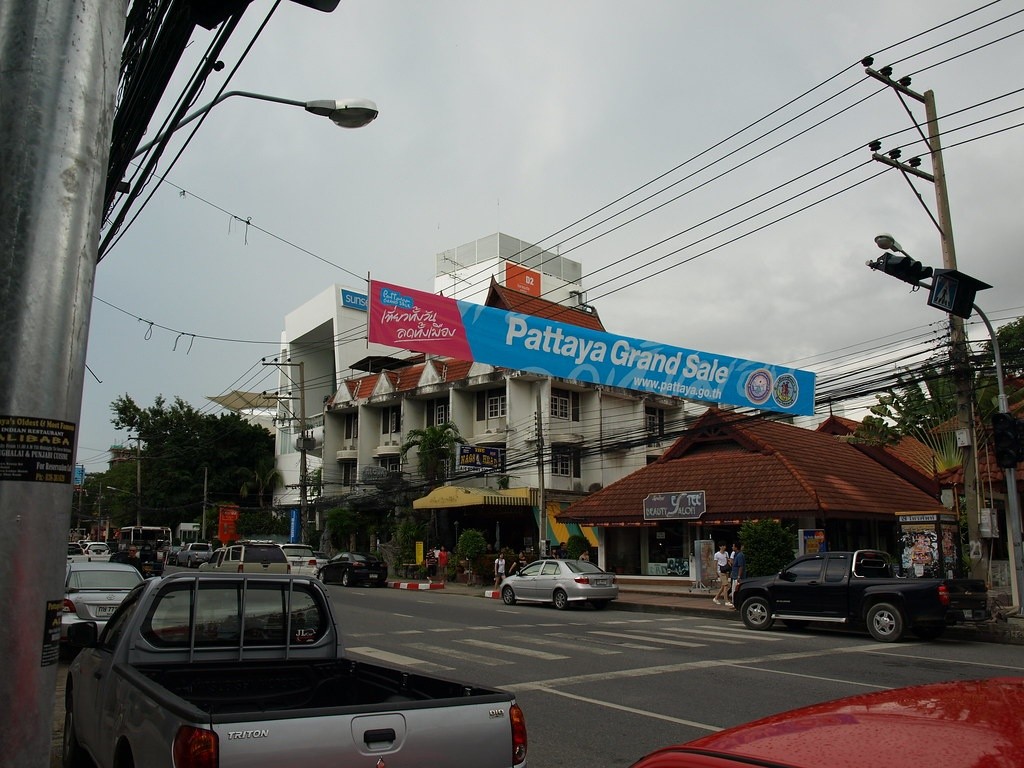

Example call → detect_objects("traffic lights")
877 253 933 284
1016 416 1024 462
992 413 1017 468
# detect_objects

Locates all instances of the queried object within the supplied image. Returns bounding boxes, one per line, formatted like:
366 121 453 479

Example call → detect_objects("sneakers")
713 598 722 604
724 602 733 606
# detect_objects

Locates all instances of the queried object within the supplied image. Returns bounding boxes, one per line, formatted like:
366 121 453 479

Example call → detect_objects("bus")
118 526 172 559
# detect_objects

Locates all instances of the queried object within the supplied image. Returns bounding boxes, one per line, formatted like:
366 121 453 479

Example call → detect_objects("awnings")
533 501 599 548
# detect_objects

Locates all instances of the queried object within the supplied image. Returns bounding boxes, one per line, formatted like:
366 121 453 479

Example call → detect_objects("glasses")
129 550 135 552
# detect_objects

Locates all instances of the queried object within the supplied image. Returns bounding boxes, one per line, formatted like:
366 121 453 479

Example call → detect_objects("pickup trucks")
733 549 992 642
62 571 528 767
175 543 215 568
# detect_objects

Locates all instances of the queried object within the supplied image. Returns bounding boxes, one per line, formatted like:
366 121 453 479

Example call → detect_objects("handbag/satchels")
497 559 499 569
720 563 732 572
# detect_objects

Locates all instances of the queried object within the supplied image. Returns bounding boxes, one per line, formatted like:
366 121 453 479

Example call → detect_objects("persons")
714 542 747 608
487 544 529 590
550 542 570 559
579 548 589 562
120 546 142 573
426 545 448 583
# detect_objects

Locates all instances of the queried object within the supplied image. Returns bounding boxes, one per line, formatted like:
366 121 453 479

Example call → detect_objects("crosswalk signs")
932 274 959 312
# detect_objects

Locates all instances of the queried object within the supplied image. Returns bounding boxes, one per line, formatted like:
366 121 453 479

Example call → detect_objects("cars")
316 552 388 588
627 677 1023 768
275 544 318 578
500 558 619 611
199 540 292 575
62 562 166 642
166 546 184 565
67 539 116 563
313 550 330 568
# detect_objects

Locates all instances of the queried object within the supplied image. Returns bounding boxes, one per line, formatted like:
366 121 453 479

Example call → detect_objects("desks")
402 564 418 579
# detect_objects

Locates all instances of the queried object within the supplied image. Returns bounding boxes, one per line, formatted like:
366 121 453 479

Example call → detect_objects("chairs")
164 605 204 641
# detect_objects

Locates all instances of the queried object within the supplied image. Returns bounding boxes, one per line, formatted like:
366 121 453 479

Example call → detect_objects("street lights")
101 90 379 226
107 486 141 526
874 232 1024 618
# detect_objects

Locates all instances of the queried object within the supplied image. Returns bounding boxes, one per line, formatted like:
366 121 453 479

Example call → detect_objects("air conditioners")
485 428 503 434
